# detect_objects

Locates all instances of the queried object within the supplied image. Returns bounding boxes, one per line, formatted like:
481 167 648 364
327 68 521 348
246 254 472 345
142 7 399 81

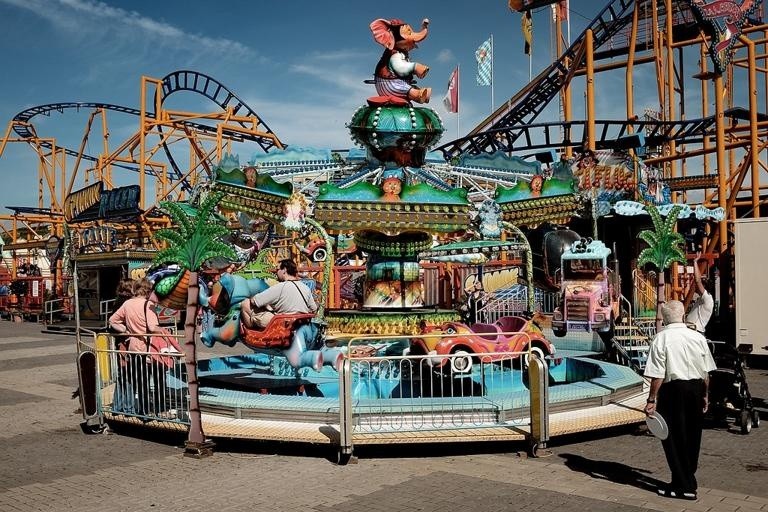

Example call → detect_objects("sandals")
657 483 698 500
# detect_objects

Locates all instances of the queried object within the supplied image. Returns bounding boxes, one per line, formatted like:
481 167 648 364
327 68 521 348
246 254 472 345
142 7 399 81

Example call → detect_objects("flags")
474 35 491 87
441 67 458 113
520 1 533 55
550 0 567 24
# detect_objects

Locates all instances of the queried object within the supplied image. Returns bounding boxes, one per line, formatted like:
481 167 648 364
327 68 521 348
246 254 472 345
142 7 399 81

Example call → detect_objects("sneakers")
141 413 179 421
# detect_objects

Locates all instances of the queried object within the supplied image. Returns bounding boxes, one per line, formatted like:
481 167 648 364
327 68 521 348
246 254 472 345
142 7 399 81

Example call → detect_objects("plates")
645 410 669 441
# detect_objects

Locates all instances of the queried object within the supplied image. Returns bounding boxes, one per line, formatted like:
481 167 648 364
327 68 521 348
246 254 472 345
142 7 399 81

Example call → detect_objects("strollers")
705 341 760 435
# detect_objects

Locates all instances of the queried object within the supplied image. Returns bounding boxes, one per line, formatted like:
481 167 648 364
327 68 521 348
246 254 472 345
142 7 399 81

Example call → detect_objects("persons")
109 278 137 418
463 280 502 328
0 281 9 296
107 277 185 424
237 258 318 330
684 251 713 335
640 299 718 502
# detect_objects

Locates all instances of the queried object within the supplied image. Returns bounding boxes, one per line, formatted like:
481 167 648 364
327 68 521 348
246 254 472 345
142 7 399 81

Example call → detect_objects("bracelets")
646 399 656 404
